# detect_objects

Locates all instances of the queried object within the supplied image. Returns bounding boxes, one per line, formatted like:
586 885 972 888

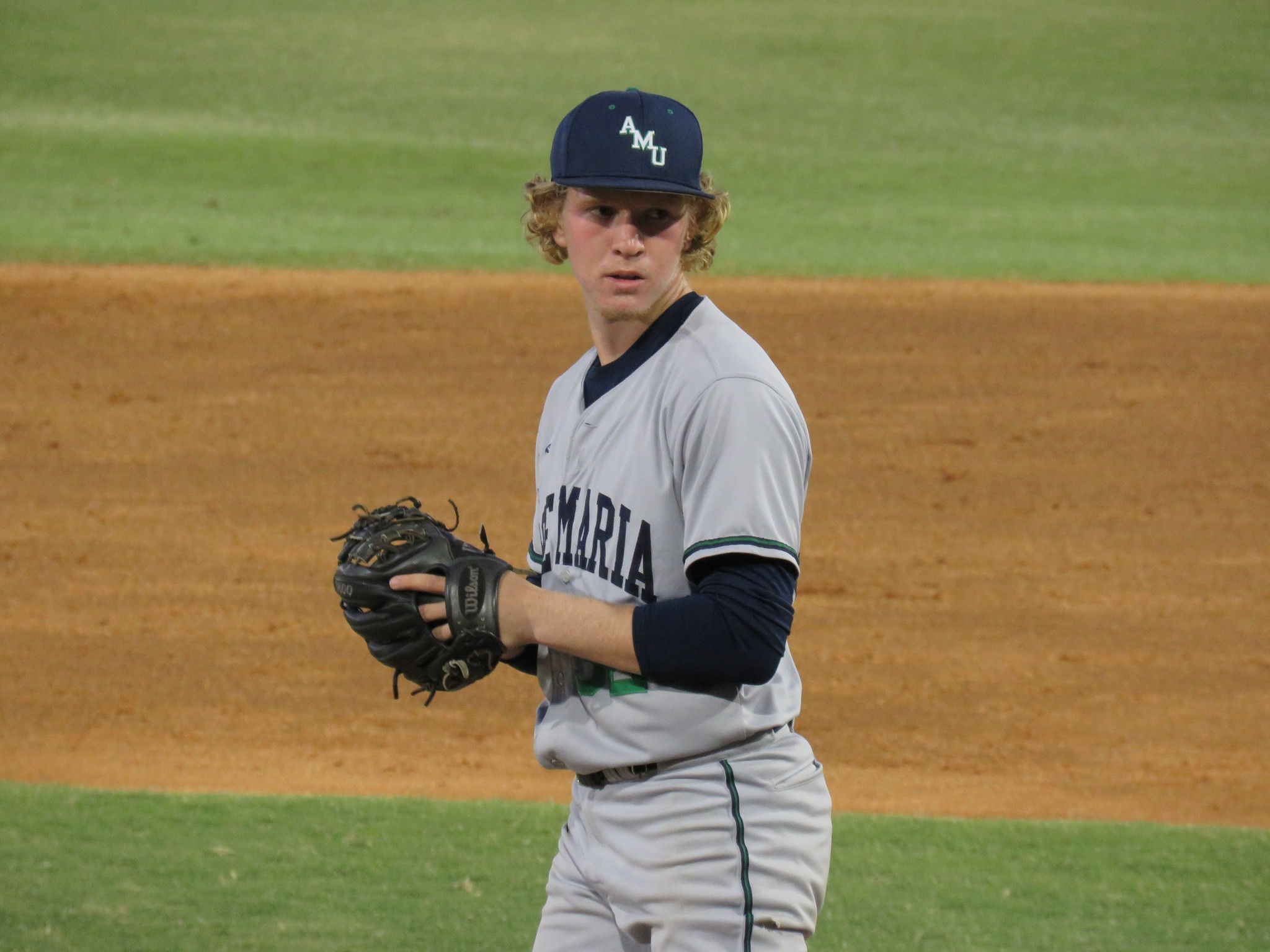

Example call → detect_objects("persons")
327 88 833 952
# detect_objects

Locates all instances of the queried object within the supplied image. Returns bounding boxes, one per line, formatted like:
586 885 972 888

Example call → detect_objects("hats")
551 88 715 201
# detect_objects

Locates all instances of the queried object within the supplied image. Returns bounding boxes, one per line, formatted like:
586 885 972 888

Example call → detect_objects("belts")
581 761 663 789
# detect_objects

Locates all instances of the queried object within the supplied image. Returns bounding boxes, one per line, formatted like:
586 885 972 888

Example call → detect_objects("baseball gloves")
332 494 513 695
